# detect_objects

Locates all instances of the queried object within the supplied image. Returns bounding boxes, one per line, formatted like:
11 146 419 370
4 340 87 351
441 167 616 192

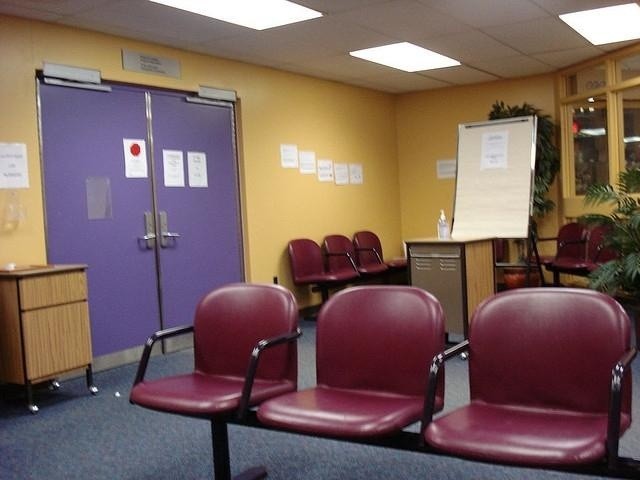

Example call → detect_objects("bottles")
437 210 450 239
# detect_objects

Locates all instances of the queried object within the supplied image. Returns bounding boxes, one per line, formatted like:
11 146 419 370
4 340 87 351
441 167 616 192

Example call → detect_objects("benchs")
127 283 640 480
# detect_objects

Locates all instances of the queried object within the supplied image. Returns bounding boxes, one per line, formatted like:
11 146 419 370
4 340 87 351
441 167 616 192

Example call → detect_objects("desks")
400 236 497 348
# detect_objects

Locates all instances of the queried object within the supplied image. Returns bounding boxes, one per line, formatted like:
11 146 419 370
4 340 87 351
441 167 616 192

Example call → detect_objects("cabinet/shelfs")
0 264 99 414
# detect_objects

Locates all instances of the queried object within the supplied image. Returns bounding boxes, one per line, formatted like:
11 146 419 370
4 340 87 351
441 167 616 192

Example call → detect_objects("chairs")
287 230 407 321
525 222 617 287
